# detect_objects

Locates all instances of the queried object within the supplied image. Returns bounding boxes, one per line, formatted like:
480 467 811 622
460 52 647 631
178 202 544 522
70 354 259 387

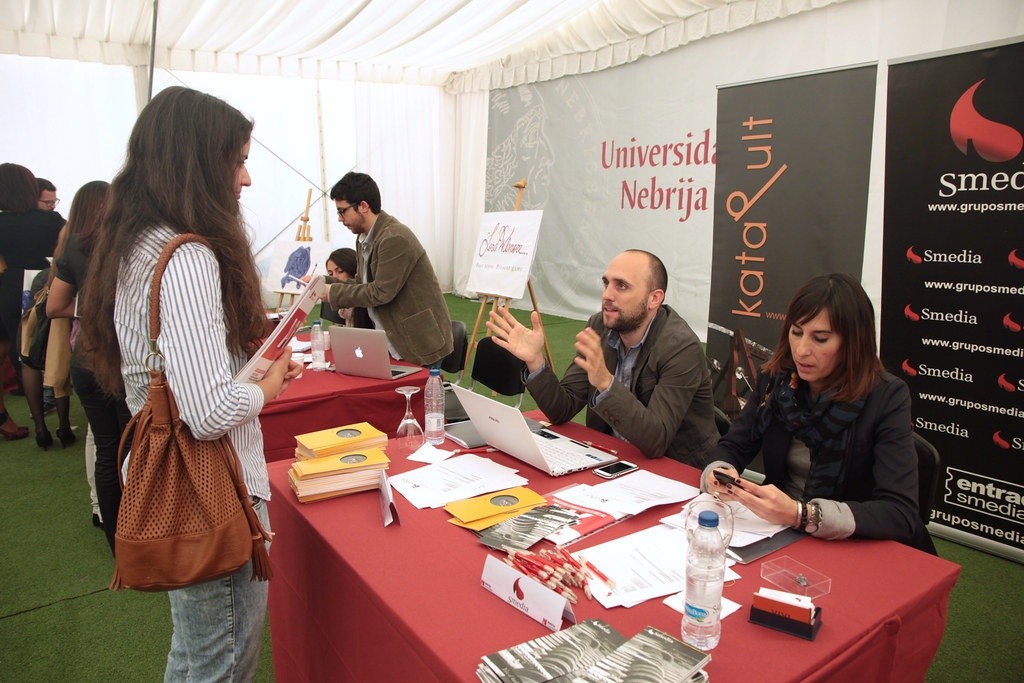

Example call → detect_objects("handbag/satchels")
19 289 54 369
109 237 273 590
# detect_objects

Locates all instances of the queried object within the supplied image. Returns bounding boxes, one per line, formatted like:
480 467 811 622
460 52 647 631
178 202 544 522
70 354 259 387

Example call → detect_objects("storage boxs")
746 604 823 642
761 556 831 601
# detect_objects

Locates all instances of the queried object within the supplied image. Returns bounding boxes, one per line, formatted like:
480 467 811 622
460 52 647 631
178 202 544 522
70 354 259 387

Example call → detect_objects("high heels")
56 428 75 448
0 410 29 437
36 430 53 451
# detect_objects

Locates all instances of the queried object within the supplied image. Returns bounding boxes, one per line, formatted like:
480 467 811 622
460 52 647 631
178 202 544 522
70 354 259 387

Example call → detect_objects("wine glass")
394 385 426 455
686 499 735 587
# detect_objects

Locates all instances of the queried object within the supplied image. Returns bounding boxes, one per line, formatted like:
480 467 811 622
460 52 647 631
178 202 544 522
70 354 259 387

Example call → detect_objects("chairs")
441 320 466 388
444 336 525 422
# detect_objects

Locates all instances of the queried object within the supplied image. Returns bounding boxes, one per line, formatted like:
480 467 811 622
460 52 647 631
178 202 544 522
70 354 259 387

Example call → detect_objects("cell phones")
592 461 640 479
714 471 744 492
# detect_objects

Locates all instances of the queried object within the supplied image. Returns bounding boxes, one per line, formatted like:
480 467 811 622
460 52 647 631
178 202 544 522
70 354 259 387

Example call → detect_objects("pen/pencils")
578 555 614 589
501 544 593 605
454 448 499 453
311 264 317 276
583 440 617 455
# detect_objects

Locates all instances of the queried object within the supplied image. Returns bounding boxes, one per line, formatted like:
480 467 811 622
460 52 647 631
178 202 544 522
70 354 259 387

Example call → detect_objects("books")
444 410 547 449
286 334 312 366
464 617 711 683
537 482 630 546
234 272 327 387
285 422 390 503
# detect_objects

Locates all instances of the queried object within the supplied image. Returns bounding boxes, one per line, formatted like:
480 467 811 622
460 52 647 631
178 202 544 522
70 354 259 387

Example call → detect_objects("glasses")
337 202 358 216
39 198 60 207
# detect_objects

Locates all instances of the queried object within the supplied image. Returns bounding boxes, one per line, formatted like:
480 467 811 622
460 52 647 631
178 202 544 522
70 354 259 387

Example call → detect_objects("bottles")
680 510 725 651
424 369 444 445
310 320 326 371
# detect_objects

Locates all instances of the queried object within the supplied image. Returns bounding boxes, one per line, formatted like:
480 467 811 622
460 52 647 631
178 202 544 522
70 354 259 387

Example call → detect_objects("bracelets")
792 500 806 532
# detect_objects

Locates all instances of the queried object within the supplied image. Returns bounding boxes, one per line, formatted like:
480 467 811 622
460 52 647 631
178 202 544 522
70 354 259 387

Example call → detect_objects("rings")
712 491 720 501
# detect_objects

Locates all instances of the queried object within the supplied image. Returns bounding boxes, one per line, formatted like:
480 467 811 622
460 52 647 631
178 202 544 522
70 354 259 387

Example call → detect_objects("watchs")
807 504 820 535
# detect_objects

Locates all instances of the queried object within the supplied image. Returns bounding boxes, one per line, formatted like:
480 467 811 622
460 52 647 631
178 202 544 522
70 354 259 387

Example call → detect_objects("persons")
698 273 939 560
486 249 723 472
326 172 455 374
88 86 301 683
0 162 140 559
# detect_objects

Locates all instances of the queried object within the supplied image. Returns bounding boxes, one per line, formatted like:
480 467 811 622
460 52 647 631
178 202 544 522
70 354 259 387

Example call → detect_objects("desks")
245 330 445 463
263 411 963 683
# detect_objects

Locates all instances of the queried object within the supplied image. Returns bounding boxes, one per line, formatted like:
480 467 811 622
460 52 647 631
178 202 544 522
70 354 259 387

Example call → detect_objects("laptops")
329 326 422 381
450 382 618 477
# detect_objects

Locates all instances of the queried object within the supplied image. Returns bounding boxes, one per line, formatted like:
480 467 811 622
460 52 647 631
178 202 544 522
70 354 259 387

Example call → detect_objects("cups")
324 330 331 351
290 352 304 379
278 307 289 320
